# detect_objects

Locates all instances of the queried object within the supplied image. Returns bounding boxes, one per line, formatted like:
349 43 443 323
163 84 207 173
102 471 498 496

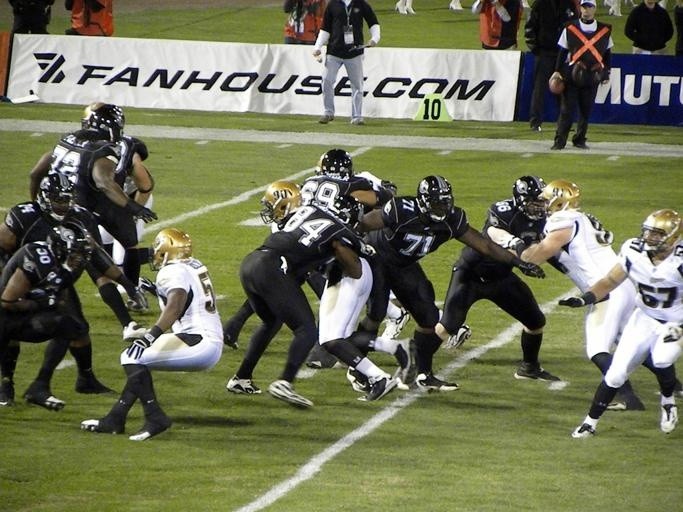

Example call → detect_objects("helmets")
512 174 581 223
416 175 454 223
36 172 77 220
45 217 96 274
259 180 304 228
148 227 194 272
82 101 126 144
314 147 365 231
640 206 682 253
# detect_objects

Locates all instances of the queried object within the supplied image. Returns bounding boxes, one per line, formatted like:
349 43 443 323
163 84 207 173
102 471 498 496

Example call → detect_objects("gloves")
519 262 545 279
559 296 586 308
23 287 59 311
125 333 155 361
663 326 683 343
122 199 159 225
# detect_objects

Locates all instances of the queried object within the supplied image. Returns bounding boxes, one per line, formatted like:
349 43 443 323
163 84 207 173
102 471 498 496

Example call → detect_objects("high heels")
395 0 416 15
449 3 463 12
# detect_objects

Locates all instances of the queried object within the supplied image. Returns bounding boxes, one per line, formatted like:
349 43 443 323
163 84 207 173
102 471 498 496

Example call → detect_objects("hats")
581 0 597 8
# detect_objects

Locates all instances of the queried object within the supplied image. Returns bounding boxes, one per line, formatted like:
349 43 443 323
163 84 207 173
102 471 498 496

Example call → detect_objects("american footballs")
549 78 565 93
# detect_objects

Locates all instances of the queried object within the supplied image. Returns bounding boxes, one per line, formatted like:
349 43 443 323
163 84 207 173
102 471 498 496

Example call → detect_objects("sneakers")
128 413 173 442
80 413 127 436
571 420 597 439
1 379 14 406
95 276 158 339
530 121 543 132
223 304 474 413
319 114 335 124
512 362 560 382
21 381 66 411
606 397 645 411
351 117 363 125
655 388 683 398
74 371 118 395
550 130 590 150
659 403 679 433
